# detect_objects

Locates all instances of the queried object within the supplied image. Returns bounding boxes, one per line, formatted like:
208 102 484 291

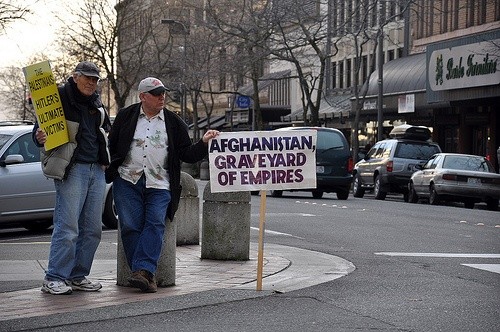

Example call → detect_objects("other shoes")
126 269 158 293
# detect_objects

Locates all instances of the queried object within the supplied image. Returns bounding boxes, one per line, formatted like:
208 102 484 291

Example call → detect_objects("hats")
74 61 101 79
138 76 170 97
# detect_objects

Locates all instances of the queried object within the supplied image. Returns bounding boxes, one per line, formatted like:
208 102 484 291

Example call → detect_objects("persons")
104 77 219 293
32 61 113 295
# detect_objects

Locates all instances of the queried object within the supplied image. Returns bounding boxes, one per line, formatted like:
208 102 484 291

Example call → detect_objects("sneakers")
65 276 102 291
41 279 73 295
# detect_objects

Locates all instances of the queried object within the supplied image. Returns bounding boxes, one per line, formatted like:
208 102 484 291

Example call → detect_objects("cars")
410 152 497 207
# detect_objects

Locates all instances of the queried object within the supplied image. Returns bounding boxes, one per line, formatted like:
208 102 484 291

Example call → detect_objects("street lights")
159 18 189 115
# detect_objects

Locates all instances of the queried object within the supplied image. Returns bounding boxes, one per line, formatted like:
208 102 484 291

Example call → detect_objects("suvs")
352 125 444 199
0 119 119 230
248 126 354 199
